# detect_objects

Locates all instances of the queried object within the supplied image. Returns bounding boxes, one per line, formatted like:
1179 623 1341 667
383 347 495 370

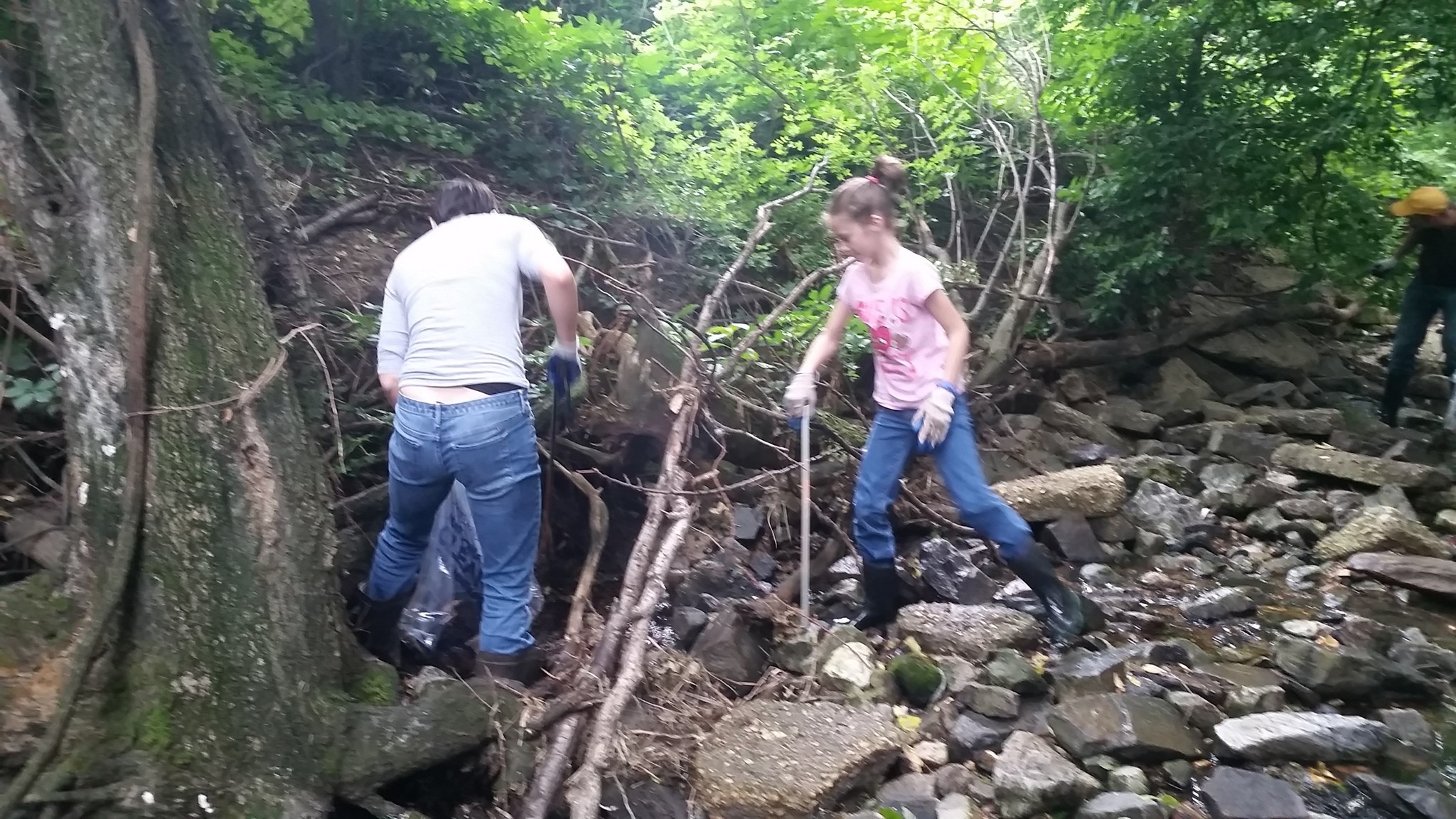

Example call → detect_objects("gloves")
911 381 958 448
1369 257 1399 278
783 372 818 419
547 334 581 390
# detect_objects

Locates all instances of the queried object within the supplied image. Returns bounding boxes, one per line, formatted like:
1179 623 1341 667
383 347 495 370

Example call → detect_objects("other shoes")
346 579 416 667
472 644 554 686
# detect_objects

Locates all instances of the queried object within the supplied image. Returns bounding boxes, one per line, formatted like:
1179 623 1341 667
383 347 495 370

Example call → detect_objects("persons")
782 154 1105 655
1369 187 1456 426
351 176 582 688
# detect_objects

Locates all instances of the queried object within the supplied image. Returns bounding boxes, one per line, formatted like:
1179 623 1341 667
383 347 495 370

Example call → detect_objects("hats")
1390 186 1448 217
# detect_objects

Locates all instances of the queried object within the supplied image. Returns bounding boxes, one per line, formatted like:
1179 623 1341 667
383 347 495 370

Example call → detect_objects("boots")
855 561 903 629
1008 537 1084 637
1379 374 1408 427
